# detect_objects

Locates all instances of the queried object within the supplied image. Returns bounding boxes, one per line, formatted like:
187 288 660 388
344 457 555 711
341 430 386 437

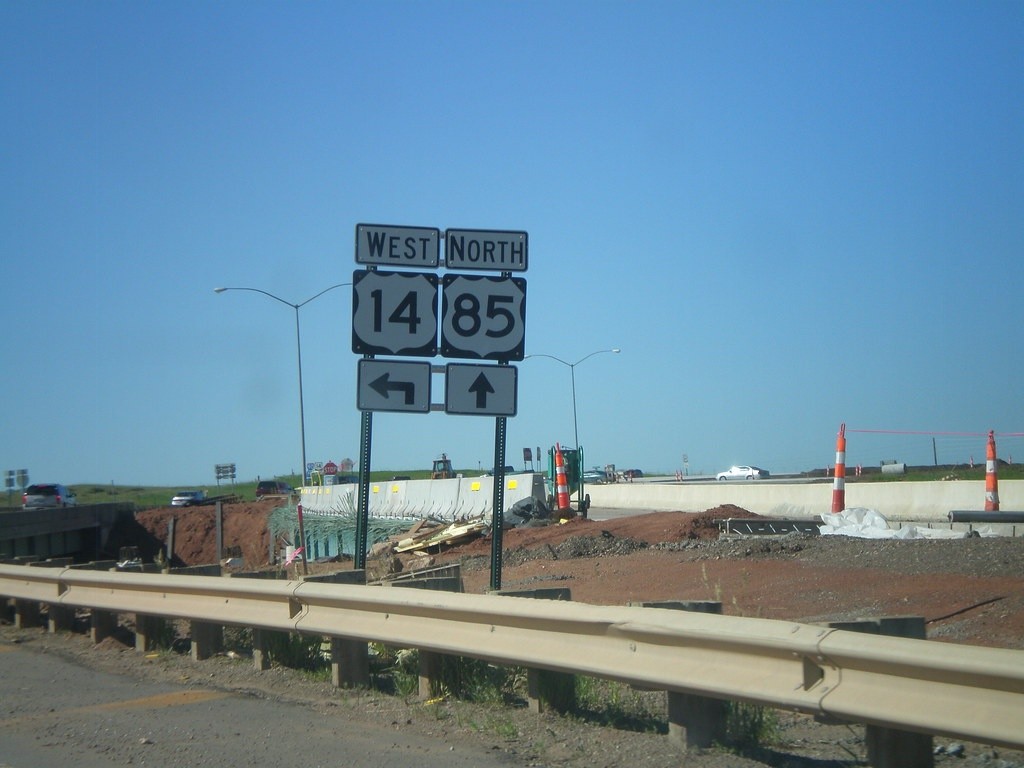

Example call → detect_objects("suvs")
256 480 297 498
21 482 77 512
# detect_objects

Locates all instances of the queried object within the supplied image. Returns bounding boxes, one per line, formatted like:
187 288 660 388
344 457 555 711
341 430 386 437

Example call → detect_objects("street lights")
215 281 353 489
524 348 621 509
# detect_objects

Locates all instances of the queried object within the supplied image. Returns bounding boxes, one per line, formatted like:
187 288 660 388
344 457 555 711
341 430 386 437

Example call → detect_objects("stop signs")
323 462 337 475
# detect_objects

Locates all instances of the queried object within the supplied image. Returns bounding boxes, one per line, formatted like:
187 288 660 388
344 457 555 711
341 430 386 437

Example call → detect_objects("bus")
429 459 456 479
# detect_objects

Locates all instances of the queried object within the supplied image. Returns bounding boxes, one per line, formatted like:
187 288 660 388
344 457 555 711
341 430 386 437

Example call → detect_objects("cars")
624 469 643 479
715 465 770 482
171 490 205 507
579 470 616 485
